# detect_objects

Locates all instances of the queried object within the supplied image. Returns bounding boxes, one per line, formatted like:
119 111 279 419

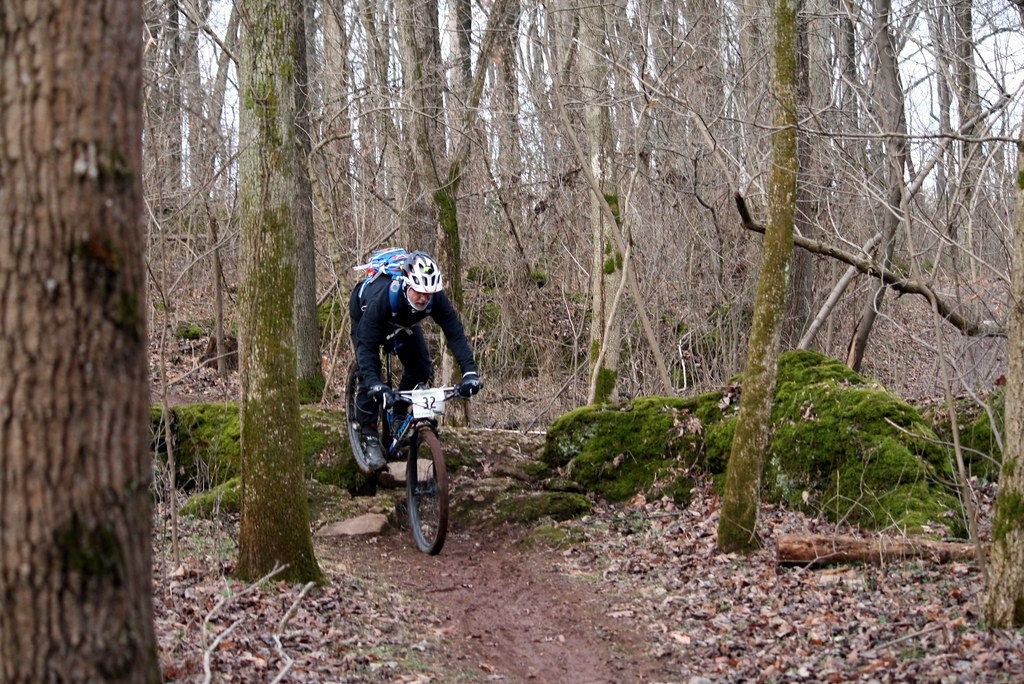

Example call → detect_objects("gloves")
459 371 481 397
371 384 391 404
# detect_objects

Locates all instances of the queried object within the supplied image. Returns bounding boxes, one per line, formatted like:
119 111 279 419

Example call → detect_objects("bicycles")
346 341 485 557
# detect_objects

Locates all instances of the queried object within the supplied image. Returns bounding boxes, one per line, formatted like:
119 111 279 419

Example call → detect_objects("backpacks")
366 246 433 317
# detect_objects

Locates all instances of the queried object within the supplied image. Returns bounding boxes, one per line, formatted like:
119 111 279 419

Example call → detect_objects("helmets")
400 251 443 293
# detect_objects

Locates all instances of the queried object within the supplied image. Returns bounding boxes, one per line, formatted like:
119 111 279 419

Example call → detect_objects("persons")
342 246 484 476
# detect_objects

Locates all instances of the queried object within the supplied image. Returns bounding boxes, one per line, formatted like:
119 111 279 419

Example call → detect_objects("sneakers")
361 435 387 468
393 393 409 422
